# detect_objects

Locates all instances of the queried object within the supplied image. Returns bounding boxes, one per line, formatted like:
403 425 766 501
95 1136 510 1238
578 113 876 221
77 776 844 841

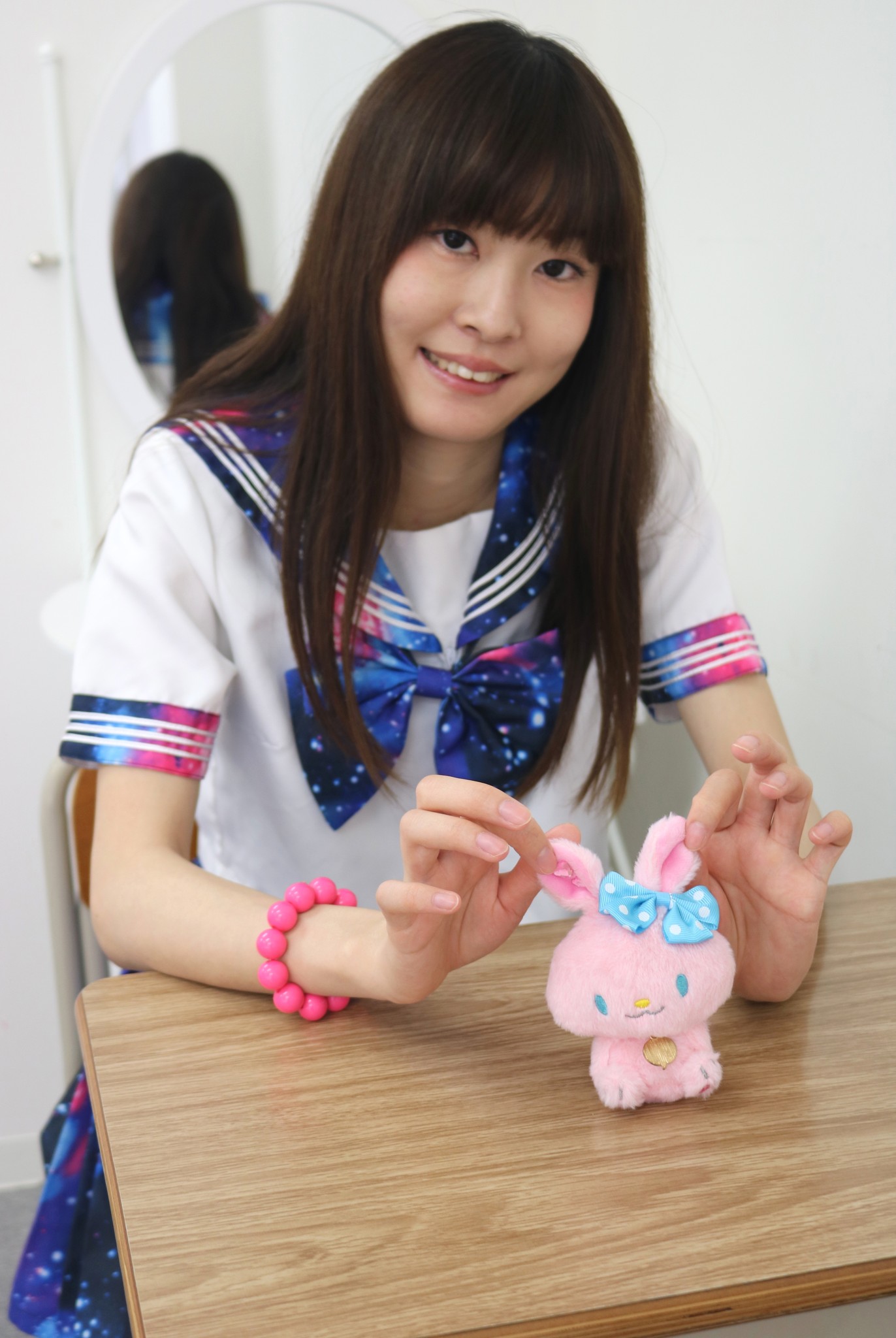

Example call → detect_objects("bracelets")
255 870 361 1021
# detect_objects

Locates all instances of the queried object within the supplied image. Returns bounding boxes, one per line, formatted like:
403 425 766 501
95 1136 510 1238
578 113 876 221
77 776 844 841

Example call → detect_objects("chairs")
60 765 208 983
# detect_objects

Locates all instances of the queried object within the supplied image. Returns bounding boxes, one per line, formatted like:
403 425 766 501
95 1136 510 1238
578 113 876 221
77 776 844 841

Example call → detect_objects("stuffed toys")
536 814 741 1111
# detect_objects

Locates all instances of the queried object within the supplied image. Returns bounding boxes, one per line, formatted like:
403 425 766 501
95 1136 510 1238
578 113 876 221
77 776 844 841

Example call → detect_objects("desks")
68 868 895 1336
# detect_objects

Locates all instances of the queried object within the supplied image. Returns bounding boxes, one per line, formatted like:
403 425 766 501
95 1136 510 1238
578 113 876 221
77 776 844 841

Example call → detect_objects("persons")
110 143 271 406
17 8 850 1338
